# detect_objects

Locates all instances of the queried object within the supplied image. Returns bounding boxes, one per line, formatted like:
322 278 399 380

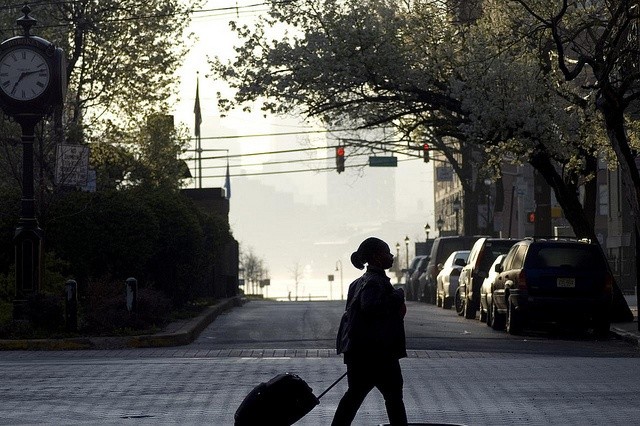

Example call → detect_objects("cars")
478 254 506 326
402 255 423 301
435 251 472 308
408 255 429 301
417 265 425 302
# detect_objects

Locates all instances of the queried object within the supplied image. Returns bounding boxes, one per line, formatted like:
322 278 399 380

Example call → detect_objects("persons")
330 238 407 425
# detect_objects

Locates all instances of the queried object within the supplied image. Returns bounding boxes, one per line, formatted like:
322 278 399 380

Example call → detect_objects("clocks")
0 48 50 101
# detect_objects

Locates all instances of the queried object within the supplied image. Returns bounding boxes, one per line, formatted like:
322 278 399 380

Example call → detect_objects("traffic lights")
336 147 344 171
423 143 429 161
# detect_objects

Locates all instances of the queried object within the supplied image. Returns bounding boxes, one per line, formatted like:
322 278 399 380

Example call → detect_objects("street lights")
404 236 409 269
425 224 430 255
335 260 343 300
396 242 400 284
453 197 461 236
436 215 444 236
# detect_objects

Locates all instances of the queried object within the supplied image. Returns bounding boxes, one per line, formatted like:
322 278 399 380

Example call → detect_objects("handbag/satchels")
336 310 350 353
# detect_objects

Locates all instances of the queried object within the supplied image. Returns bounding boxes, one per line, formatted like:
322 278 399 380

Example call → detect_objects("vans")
424 235 491 304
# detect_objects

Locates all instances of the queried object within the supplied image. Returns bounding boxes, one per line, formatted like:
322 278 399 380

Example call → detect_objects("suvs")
453 237 525 319
490 236 613 340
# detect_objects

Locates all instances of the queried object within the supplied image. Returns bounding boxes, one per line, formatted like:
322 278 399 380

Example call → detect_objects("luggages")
234 370 348 426
